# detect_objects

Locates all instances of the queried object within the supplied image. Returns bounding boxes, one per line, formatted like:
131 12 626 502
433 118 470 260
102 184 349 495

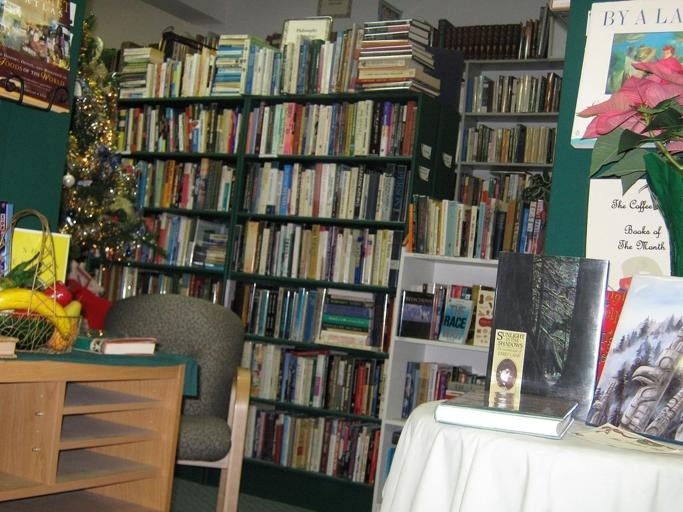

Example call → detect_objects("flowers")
577 54 683 197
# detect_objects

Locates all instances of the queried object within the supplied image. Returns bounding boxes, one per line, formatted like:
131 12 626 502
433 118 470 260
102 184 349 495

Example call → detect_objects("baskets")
1 209 84 354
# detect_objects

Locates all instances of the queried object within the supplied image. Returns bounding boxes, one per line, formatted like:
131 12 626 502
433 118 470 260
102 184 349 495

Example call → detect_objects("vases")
641 153 683 277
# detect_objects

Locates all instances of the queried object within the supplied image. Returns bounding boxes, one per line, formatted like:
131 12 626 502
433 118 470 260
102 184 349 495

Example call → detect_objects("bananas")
0 288 71 341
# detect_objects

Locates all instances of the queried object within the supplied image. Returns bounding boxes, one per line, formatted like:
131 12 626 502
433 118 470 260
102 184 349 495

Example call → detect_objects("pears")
62 294 84 315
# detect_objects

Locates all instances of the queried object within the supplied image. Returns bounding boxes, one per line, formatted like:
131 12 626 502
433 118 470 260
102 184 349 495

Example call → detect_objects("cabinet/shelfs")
369 253 498 512
91 16 462 503
0 334 197 512
454 56 565 202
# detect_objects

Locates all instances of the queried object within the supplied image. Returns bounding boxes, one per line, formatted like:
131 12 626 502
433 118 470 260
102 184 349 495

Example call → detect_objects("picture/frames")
318 0 351 17
378 1 404 22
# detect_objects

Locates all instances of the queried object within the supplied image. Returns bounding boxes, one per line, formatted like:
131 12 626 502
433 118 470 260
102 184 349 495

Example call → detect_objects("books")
357 18 441 98
112 102 243 152
115 213 228 268
242 341 388 418
415 171 548 260
247 162 410 222
247 99 415 156
240 16 364 97
230 222 403 286
94 264 237 310
245 286 392 348
119 31 249 98
467 123 554 164
445 20 547 60
469 71 563 114
401 362 485 423
244 402 383 484
433 389 580 439
120 158 235 211
396 280 496 346
74 331 158 356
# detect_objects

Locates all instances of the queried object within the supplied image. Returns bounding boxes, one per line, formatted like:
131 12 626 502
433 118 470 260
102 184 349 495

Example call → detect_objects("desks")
385 394 683 512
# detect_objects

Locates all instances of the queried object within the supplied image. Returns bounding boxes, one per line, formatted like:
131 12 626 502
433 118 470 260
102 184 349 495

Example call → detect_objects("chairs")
93 294 253 512
